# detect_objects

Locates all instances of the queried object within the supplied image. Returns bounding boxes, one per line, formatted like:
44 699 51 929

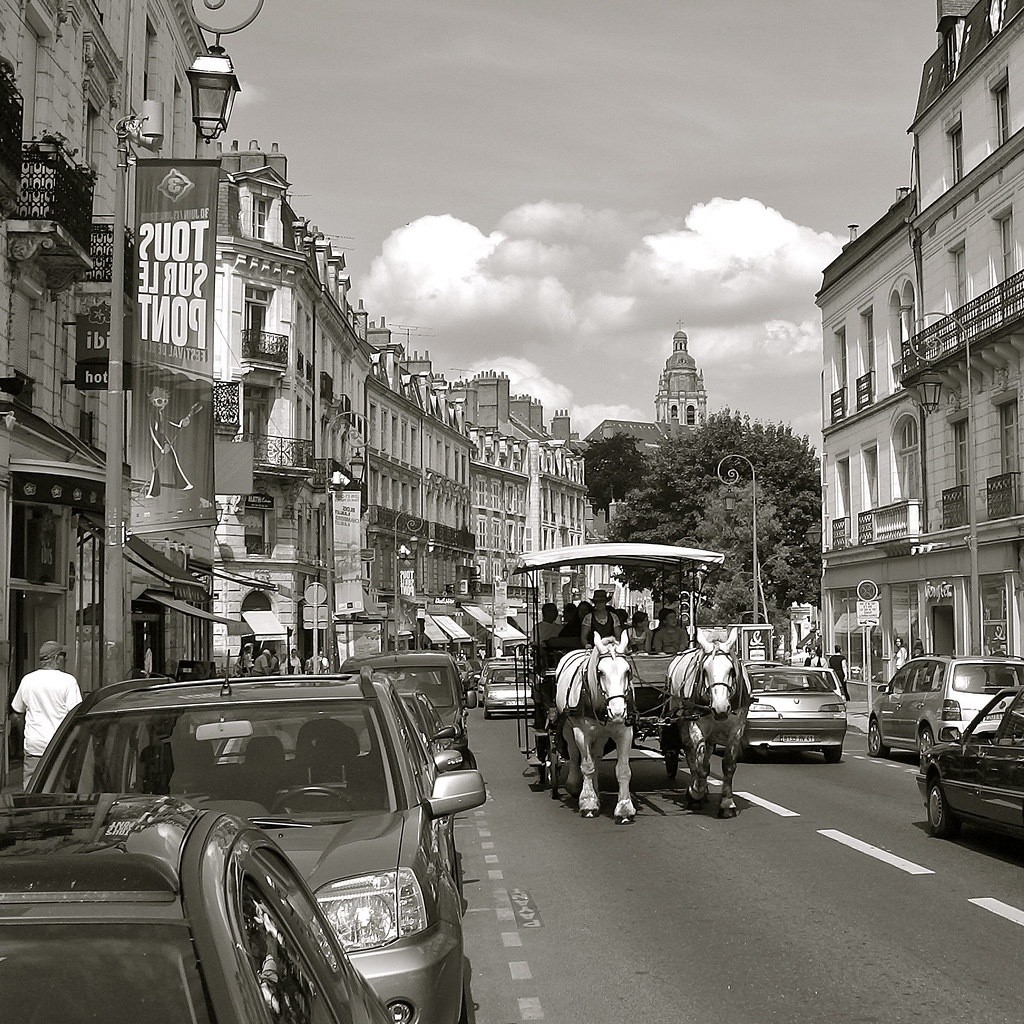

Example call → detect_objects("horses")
664 626 752 819
554 628 637 825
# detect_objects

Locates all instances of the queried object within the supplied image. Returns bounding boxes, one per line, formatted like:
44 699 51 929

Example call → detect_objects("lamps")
138 537 194 558
911 543 945 555
0 411 16 431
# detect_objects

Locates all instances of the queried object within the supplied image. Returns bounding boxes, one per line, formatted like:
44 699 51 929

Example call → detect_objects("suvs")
867 653 1024 774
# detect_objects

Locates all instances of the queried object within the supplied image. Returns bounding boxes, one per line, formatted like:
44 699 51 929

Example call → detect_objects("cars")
916 686 1024 867
340 651 477 770
1 795 397 1024
484 667 536 721
457 656 532 708
810 669 846 706
713 668 848 765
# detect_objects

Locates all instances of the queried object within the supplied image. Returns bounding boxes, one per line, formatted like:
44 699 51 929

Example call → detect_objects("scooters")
26 673 488 1024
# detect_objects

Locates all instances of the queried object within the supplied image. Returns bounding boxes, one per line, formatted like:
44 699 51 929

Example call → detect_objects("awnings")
420 615 472 644
797 633 822 649
145 592 253 635
462 606 537 645
358 589 382 619
81 512 211 603
239 611 287 640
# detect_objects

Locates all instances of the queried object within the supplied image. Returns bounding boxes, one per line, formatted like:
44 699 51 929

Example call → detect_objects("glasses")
59 651 66 657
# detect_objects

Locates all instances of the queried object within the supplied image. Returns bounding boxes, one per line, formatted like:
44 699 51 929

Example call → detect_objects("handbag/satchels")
241 667 249 674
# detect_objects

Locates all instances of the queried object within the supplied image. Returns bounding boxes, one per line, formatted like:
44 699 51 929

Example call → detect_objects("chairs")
282 719 364 813
146 737 214 797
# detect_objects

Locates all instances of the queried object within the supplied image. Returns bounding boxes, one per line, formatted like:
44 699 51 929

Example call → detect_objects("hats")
658 609 675 620
588 590 612 602
263 649 271 655
39 641 67 661
632 611 647 623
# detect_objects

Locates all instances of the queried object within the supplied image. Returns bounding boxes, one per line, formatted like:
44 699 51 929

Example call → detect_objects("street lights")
395 510 424 649
717 455 760 623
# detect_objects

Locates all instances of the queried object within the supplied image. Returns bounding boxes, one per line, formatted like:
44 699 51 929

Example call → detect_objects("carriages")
511 543 753 825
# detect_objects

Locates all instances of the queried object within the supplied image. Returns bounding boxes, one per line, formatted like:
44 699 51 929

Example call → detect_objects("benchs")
536 635 583 675
213 737 296 799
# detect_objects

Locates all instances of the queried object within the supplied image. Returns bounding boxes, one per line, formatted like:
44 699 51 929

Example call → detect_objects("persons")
530 590 689 655
496 646 503 656
241 646 330 675
829 645 850 702
895 637 924 671
9 640 83 792
802 646 827 667
